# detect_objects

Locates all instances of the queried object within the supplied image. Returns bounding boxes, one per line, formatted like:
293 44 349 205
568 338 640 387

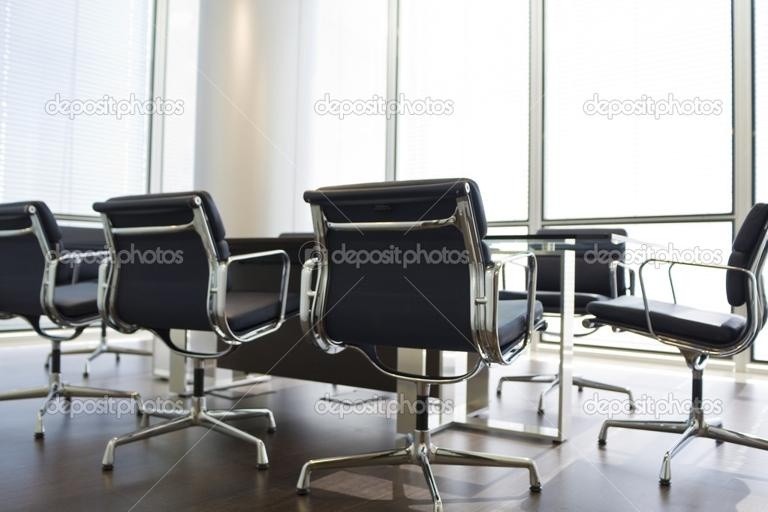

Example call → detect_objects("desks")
150 234 572 444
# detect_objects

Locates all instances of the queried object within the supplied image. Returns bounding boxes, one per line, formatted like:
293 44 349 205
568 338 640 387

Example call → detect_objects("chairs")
498 227 636 415
92 189 300 472
44 213 153 379
1 199 145 440
583 199 767 487
296 178 542 511
